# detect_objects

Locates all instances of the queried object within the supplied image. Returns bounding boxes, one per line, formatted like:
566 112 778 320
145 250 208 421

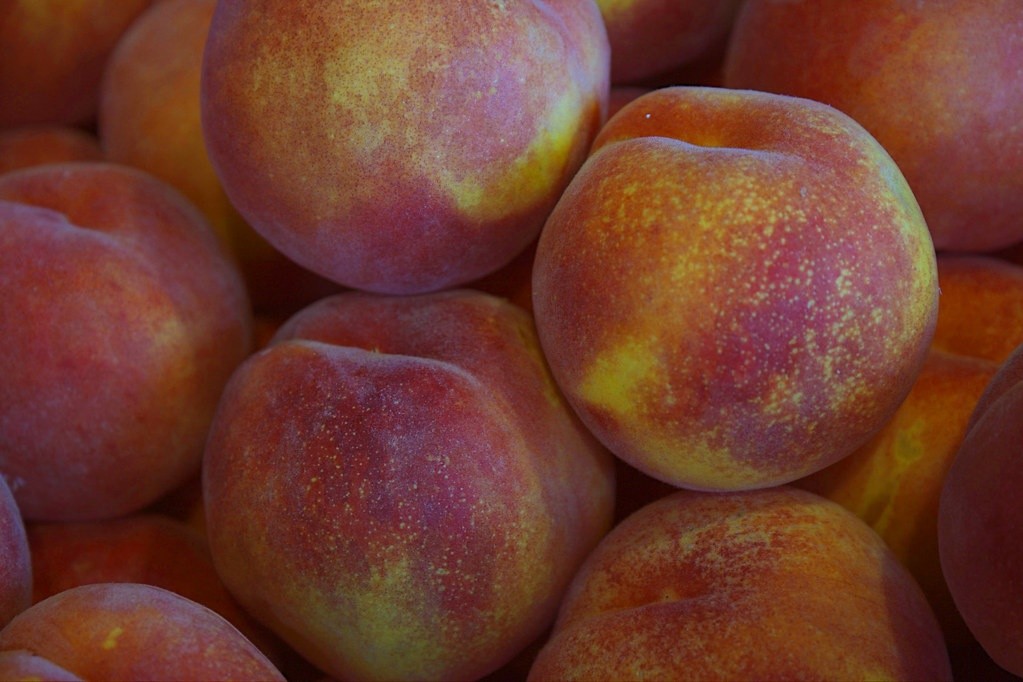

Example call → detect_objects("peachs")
0 0 1023 682
